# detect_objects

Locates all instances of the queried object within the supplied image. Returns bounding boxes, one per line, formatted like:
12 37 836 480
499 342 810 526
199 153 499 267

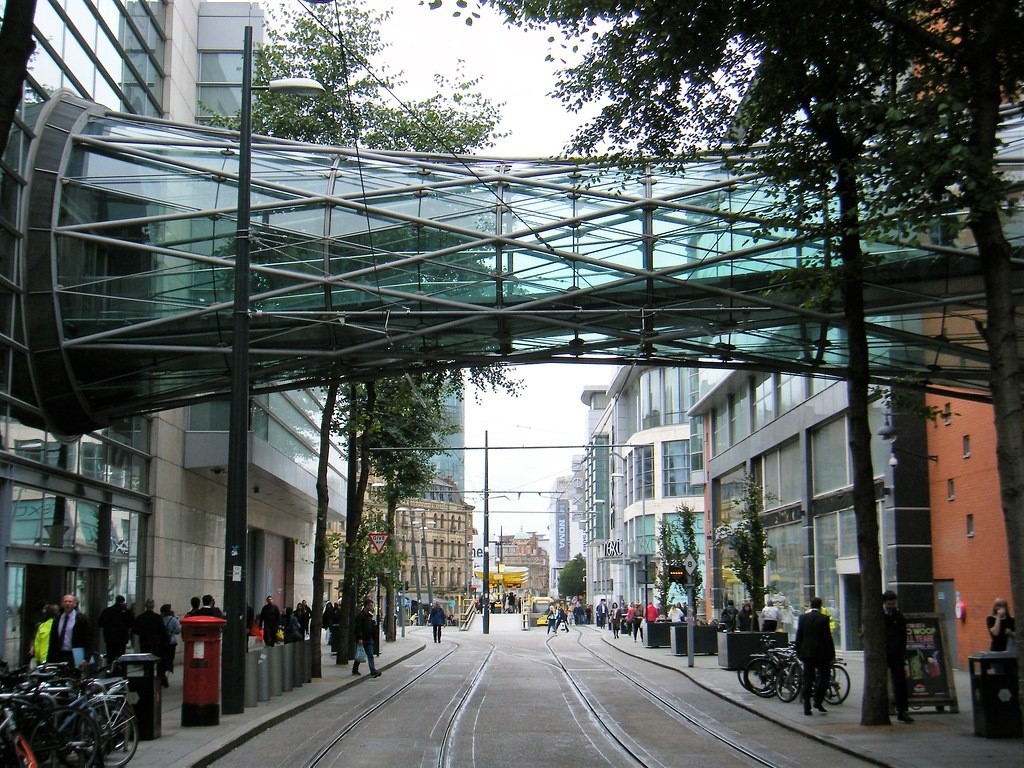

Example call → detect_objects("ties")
58 614 70 656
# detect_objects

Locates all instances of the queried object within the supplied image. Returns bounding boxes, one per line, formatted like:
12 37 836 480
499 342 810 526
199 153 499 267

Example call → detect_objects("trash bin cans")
620 614 628 633
967 652 1024 738
116 653 163 740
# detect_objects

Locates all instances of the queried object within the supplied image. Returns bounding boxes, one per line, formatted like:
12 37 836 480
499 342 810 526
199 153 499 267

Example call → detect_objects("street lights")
419 527 428 588
609 472 646 554
637 553 655 622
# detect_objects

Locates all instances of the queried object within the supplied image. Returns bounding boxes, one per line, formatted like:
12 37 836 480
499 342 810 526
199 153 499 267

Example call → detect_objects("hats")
435 602 439 606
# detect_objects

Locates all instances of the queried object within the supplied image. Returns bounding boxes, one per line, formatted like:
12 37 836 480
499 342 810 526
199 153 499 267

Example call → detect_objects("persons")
26 594 397 677
721 600 739 632
430 601 445 643
410 611 456 626
797 597 836 715
805 604 836 635
737 602 760 632
545 605 557 636
986 598 1016 673
474 591 526 613
564 599 686 642
761 600 779 632
553 606 569 633
776 601 810 638
882 590 915 722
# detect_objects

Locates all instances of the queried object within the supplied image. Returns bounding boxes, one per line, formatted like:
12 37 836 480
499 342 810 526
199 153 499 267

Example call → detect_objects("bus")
530 596 554 626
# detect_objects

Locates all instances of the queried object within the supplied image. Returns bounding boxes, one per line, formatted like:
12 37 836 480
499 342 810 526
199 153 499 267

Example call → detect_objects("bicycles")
737 635 852 704
0 661 139 768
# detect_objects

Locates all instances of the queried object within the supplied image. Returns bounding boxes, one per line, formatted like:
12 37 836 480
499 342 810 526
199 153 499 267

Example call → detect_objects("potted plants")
643 503 718 654
715 472 788 668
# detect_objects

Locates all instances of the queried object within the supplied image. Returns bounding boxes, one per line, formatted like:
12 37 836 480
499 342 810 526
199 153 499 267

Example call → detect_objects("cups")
925 658 939 676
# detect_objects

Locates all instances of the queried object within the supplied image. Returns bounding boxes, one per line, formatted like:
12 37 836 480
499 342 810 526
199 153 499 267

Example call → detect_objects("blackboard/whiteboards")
888 611 956 705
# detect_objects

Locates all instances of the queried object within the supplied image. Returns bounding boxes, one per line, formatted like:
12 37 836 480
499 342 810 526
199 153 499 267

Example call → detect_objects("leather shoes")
897 711 914 723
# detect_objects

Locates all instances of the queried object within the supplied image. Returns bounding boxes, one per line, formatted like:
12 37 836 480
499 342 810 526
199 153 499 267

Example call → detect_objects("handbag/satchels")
639 618 644 629
251 624 264 640
354 642 366 664
549 613 556 620
276 628 285 640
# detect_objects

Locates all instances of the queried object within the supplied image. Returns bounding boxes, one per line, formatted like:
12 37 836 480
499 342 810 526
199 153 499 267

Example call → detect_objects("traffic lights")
337 579 344 599
668 566 686 583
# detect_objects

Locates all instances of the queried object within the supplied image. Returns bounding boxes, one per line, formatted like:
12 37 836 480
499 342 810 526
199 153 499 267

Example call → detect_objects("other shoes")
804 709 812 716
371 669 381 678
813 702 827 712
351 668 361 675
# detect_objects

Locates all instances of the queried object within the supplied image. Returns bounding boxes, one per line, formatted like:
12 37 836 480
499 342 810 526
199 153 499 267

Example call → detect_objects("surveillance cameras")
889 457 898 468
214 469 223 475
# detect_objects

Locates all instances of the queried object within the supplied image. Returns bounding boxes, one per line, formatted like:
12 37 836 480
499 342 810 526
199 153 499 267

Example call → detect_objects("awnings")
402 592 449 605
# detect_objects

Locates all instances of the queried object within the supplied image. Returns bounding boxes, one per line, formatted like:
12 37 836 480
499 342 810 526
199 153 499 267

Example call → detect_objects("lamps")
876 409 937 439
610 473 624 477
595 499 605 503
